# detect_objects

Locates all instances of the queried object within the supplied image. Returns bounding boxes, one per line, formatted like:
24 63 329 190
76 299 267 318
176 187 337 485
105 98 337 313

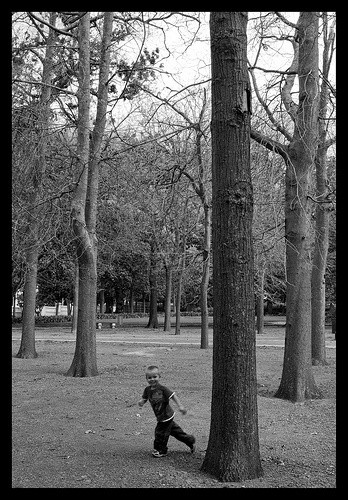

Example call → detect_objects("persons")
138 366 195 458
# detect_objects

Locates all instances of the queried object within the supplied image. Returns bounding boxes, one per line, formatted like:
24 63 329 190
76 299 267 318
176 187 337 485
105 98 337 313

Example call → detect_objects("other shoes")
152 450 168 457
190 436 196 456
